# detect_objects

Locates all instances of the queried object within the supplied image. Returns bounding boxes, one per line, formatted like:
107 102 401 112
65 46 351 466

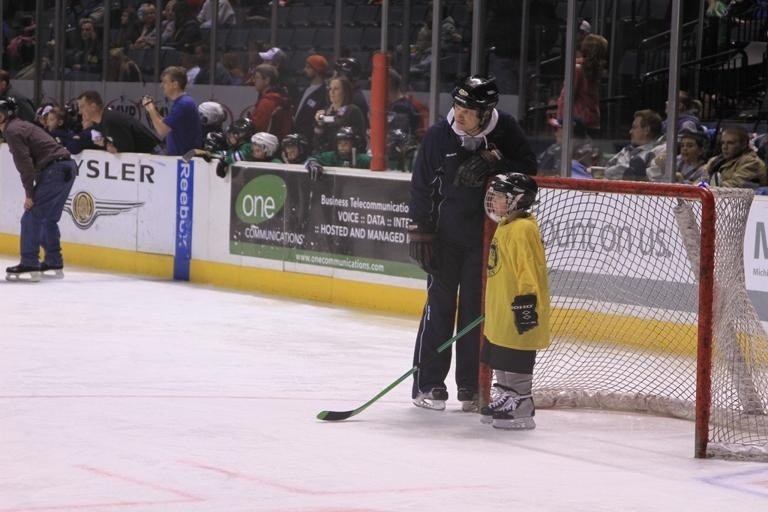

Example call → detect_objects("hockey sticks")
317 314 486 421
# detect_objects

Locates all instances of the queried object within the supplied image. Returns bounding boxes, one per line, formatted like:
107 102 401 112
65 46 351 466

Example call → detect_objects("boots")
481 384 535 419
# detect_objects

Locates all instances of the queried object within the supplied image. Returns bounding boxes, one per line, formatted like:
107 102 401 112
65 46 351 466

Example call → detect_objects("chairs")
527 99 768 197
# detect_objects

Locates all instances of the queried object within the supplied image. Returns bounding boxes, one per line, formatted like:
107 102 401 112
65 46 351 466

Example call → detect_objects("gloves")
408 222 443 275
453 148 504 187
203 148 213 162
511 295 538 334
307 160 323 182
216 160 228 178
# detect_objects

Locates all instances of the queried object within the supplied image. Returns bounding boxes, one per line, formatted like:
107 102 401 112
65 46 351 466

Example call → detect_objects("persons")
2 0 766 188
480 171 552 419
408 74 537 401
0 96 79 273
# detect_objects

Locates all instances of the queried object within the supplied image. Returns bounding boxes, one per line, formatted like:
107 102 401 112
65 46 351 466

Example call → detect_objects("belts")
41 157 69 171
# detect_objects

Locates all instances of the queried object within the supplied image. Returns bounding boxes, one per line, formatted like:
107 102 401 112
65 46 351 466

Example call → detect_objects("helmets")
0 95 17 119
228 117 256 142
451 74 499 127
197 101 223 126
335 125 363 160
282 133 309 163
484 173 537 223
335 58 362 80
251 132 278 159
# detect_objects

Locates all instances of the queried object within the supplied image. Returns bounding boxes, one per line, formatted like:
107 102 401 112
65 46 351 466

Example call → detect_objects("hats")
561 19 591 33
307 55 329 74
548 116 584 132
259 48 287 65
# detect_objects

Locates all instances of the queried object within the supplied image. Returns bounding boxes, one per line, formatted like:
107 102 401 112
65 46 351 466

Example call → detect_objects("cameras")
318 114 335 123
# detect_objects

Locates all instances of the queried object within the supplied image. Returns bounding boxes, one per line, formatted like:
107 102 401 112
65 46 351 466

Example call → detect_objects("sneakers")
457 385 479 401
6 262 63 272
417 383 448 400
